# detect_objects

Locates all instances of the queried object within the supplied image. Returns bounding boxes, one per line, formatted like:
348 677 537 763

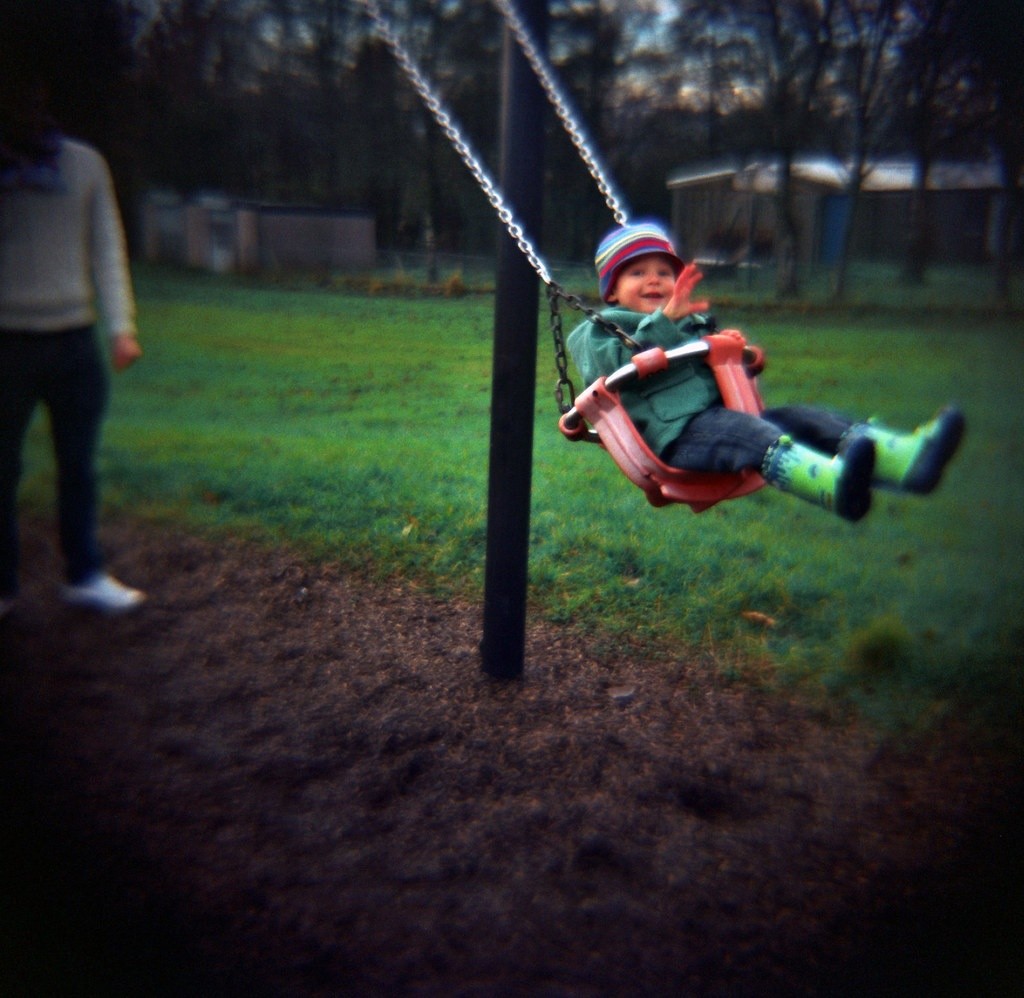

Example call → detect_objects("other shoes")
58 572 148 612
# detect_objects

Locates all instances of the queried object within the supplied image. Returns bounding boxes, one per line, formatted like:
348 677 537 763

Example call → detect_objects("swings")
359 0 773 514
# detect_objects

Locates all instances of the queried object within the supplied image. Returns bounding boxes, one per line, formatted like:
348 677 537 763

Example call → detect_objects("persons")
0 70 149 615
565 224 965 522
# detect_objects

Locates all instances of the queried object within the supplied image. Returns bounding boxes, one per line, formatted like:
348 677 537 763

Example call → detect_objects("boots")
837 403 966 492
760 434 875 522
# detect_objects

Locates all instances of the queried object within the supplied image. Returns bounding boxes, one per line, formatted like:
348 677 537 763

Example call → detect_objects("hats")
594 222 685 305
